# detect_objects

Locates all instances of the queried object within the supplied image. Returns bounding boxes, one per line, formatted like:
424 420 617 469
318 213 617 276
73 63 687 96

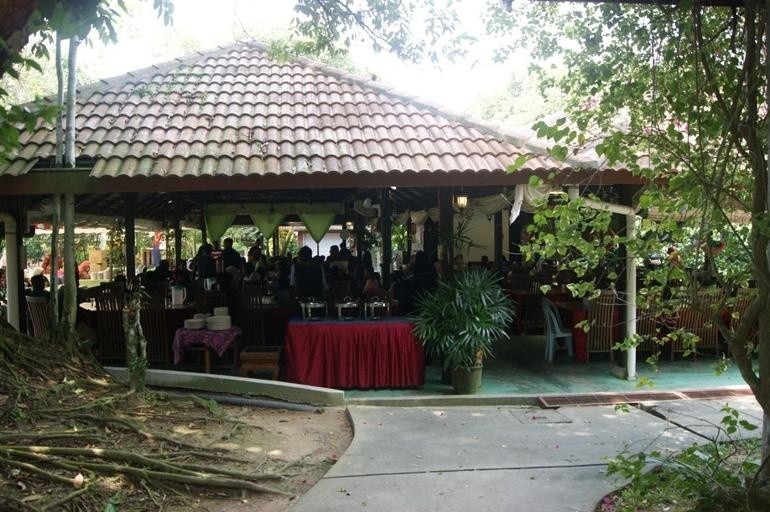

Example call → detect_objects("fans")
351 196 381 219
24 196 66 236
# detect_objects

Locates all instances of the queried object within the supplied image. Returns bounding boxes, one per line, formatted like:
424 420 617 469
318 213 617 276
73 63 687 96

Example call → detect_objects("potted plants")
405 264 517 394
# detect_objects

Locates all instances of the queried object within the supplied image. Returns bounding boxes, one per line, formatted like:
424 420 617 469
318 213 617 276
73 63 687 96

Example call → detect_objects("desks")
239 344 282 380
175 323 242 375
283 317 427 389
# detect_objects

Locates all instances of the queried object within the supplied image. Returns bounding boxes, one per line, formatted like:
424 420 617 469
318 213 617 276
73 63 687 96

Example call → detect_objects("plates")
183 315 231 331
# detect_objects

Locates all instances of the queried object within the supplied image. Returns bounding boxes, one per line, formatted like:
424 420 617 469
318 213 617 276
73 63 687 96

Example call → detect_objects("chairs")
520 275 760 365
24 262 391 367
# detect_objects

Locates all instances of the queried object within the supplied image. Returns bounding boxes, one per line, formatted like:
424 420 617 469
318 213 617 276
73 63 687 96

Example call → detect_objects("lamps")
456 185 472 217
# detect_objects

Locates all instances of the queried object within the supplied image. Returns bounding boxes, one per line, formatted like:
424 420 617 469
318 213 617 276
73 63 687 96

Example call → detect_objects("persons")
510 225 542 267
240 244 438 319
114 275 133 299
402 222 426 262
666 246 678 266
24 275 51 336
480 256 489 267
208 272 241 342
643 257 660 270
154 237 241 302
